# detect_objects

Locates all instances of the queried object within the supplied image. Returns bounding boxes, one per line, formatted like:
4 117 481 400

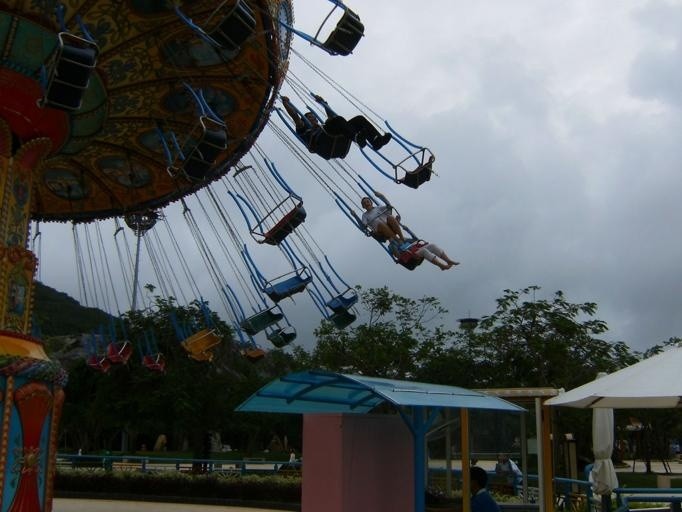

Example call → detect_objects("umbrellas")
588 371 621 511
542 346 682 409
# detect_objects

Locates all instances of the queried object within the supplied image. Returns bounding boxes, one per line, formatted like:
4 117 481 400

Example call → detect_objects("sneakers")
355 132 367 149
372 132 393 151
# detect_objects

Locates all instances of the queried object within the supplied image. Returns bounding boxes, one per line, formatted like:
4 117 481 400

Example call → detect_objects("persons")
387 236 461 271
469 465 502 511
289 448 296 462
280 95 393 161
494 446 523 485
350 191 407 245
